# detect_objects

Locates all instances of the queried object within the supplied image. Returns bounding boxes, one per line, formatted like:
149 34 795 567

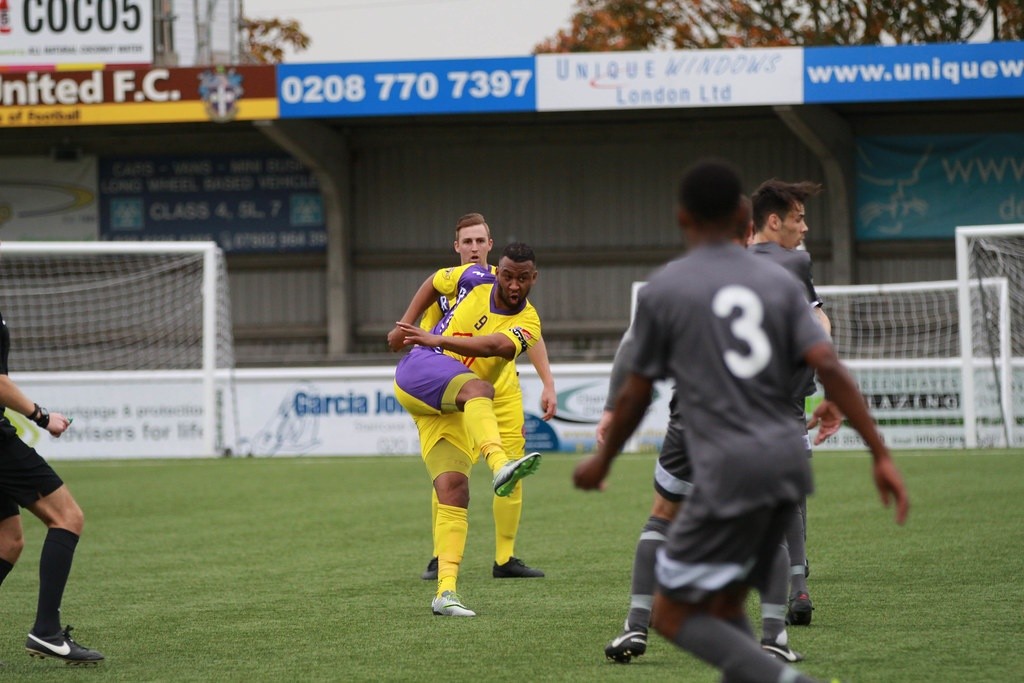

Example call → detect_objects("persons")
387 214 559 618
0 312 105 666
570 159 910 683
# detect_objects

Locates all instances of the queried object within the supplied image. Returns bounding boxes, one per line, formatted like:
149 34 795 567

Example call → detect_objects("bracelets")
26 403 39 420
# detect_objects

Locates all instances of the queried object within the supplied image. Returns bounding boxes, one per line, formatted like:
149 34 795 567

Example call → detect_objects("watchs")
37 406 49 427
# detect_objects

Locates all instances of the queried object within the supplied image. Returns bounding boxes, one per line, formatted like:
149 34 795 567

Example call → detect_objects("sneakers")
493 452 542 497
492 557 545 578
25 624 105 666
761 638 806 664
785 590 814 626
605 628 648 663
430 589 476 618
421 555 439 579
